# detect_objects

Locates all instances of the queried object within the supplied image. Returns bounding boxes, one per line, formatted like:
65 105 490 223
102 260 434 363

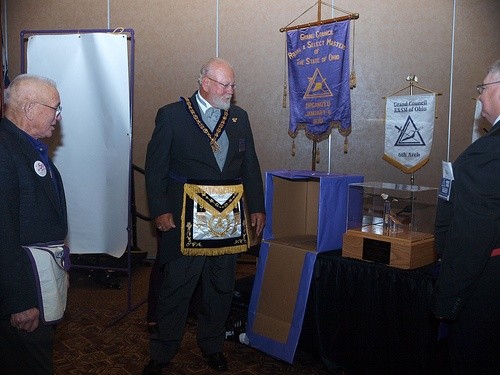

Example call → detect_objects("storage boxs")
346 182 440 241
245 170 364 364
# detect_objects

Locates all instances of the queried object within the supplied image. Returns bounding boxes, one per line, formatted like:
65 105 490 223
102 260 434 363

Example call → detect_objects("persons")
141 57 268 375
0 73 71 375
429 58 500 375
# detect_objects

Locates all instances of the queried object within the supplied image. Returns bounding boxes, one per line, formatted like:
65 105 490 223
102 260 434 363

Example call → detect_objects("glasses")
25 96 62 116
205 75 237 88
476 81 500 94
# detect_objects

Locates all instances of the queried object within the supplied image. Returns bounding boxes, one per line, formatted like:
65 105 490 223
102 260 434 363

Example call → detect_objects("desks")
247 245 443 375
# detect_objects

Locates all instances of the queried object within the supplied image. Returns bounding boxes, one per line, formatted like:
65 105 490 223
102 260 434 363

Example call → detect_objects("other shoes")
206 351 227 371
143 359 162 375
